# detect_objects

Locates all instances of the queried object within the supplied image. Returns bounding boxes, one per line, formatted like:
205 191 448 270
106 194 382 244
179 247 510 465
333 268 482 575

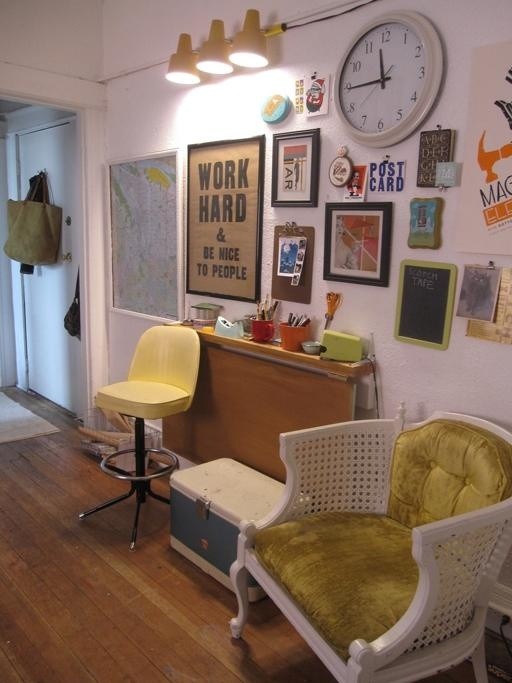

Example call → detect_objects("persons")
347 170 361 194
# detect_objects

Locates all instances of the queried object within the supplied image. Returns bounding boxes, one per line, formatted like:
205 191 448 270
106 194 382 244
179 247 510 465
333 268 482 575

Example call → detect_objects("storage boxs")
169 457 310 604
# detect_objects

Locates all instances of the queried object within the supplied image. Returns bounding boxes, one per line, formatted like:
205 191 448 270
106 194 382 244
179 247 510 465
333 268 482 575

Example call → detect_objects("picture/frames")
272 128 320 206
103 148 186 325
323 201 392 286
186 135 265 302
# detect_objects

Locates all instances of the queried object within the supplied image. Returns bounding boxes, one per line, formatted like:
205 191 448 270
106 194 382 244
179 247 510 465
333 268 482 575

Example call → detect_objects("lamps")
166 9 268 86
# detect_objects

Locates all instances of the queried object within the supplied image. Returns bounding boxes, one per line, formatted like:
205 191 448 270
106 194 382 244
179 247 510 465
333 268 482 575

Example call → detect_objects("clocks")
334 11 443 147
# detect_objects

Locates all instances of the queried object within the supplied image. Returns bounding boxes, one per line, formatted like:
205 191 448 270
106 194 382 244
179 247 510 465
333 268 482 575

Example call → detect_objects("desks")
162 319 376 483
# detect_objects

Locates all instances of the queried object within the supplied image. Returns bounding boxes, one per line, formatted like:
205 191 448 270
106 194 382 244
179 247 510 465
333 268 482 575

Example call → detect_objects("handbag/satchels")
64 266 81 340
2 172 63 266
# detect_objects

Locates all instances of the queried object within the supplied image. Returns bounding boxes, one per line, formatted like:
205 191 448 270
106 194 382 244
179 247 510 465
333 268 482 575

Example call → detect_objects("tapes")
225 323 232 327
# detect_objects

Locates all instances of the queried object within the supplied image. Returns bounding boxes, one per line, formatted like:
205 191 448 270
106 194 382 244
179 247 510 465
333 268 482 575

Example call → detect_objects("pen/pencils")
257 294 278 321
289 313 312 328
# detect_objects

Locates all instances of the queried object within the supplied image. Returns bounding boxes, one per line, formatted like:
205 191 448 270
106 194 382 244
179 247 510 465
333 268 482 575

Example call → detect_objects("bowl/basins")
302 341 319 354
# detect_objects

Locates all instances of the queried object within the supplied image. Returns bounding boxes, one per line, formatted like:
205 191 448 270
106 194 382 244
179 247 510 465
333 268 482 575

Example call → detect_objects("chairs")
79 324 201 550
230 401 512 683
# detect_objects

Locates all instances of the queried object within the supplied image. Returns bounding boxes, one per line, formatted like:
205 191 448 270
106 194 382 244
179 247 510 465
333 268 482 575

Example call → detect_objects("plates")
329 156 351 185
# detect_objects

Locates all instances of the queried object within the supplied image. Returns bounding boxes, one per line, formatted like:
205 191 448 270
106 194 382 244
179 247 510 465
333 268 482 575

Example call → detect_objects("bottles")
415 205 427 226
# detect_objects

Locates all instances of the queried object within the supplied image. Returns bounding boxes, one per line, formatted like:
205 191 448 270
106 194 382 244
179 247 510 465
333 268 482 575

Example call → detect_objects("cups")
279 322 313 352
249 317 275 341
